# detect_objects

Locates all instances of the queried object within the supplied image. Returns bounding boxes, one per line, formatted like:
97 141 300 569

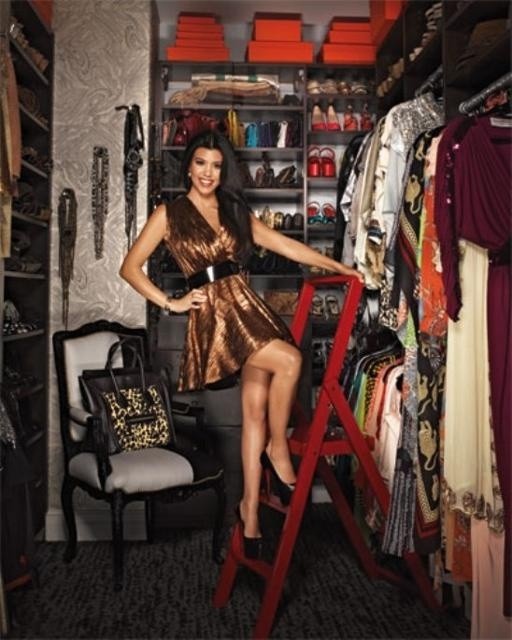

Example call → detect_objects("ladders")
209 273 445 639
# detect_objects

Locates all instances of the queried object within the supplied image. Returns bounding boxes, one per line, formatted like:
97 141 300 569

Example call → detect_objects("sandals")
310 337 334 374
307 200 337 225
310 294 341 321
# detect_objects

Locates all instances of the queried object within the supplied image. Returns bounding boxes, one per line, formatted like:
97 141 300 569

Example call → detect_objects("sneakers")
409 3 443 62
376 57 404 98
307 78 375 96
9 14 50 69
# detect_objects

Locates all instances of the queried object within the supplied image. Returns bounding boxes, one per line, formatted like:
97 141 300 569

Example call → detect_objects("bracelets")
164 295 172 315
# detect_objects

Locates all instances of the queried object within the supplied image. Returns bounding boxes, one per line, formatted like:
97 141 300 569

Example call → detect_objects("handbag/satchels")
264 287 300 316
249 235 304 274
255 166 302 188
260 206 303 230
162 110 303 148
163 152 184 186
78 365 177 452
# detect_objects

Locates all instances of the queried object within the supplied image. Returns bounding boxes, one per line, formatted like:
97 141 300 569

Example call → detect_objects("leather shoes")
327 105 340 131
312 103 326 131
343 104 358 131
361 104 374 130
309 246 335 277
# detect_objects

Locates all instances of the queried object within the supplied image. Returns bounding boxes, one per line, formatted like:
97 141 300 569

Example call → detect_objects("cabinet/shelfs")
152 61 377 429
373 1 511 127
0 0 58 593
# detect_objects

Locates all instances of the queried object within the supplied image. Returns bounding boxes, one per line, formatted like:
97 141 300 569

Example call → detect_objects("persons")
119 129 365 580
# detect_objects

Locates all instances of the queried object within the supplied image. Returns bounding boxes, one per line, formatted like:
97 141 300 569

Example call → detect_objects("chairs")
53 318 231 594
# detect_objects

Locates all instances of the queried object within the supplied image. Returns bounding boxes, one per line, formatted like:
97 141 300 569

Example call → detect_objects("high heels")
234 496 265 560
260 450 296 505
308 147 335 177
